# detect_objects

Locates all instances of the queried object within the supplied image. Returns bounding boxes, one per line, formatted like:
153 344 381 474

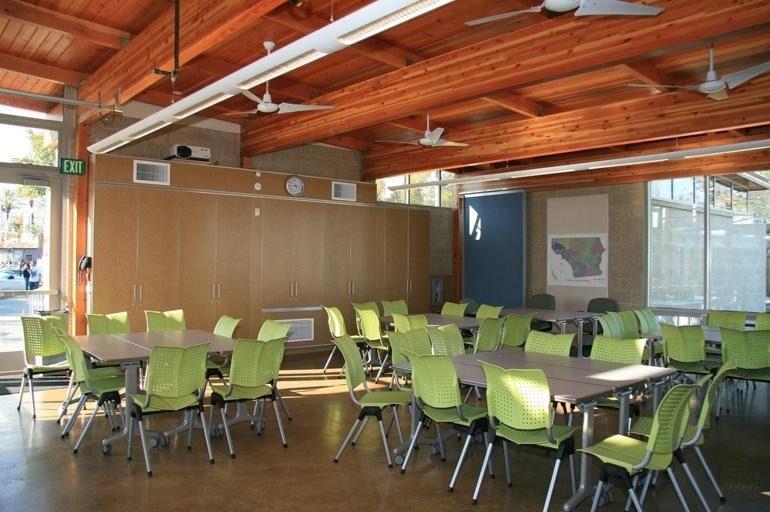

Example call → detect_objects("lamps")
85 0 455 156
387 136 770 191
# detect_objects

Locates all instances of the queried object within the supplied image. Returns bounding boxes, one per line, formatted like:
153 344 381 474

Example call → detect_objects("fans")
221 40 337 117
374 111 471 148
626 49 770 101
464 0 665 27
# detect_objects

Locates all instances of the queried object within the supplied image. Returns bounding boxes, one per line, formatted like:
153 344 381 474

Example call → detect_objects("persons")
0 259 42 291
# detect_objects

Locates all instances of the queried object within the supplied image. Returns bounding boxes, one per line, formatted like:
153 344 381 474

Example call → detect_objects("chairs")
48 322 133 456
212 337 290 459
222 318 291 423
319 293 770 511
123 341 216 475
80 310 132 410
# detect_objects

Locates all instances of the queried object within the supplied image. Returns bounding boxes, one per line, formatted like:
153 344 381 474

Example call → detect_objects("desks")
61 327 248 454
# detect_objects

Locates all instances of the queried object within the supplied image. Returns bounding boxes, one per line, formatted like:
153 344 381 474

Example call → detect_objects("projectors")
170 144 211 162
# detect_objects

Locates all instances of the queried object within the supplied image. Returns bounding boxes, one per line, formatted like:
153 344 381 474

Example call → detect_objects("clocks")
284 174 304 197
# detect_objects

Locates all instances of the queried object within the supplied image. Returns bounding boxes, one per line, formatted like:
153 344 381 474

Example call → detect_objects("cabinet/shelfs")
85 183 454 356
